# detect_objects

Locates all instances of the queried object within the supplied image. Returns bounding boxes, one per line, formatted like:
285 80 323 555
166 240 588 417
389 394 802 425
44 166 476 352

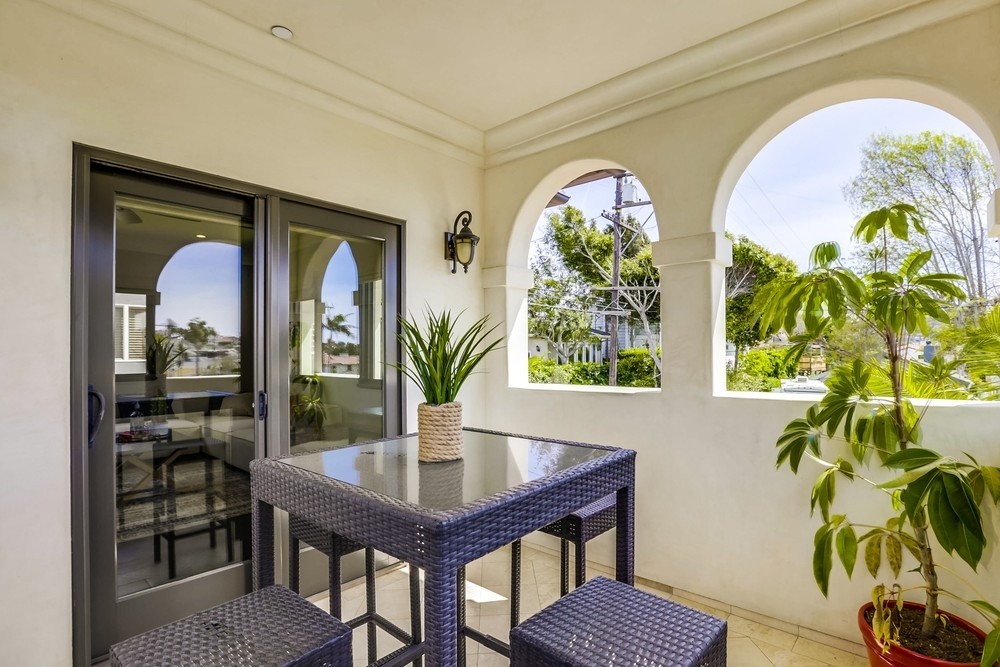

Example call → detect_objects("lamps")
445 211 480 274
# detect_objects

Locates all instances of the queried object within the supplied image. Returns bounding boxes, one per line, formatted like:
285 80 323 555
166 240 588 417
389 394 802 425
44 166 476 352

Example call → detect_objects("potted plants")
144 334 194 424
289 311 355 447
378 300 509 463
749 204 1000 667
146 388 170 435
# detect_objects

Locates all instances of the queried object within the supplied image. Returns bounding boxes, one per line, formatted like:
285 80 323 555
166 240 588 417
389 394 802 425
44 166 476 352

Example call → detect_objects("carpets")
117 458 250 543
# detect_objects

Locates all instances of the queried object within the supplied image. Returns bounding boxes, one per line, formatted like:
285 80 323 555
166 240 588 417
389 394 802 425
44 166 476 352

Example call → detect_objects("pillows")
218 392 255 416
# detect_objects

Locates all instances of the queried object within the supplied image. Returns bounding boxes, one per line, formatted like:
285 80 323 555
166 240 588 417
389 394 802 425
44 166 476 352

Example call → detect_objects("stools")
108 491 728 667
152 438 236 579
116 427 177 532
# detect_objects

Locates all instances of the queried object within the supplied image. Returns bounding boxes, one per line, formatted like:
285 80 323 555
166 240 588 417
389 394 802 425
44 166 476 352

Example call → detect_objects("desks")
116 389 231 419
349 407 382 444
250 426 637 667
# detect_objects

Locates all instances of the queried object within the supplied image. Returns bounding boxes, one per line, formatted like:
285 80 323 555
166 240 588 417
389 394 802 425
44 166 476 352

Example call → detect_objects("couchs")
116 409 374 474
